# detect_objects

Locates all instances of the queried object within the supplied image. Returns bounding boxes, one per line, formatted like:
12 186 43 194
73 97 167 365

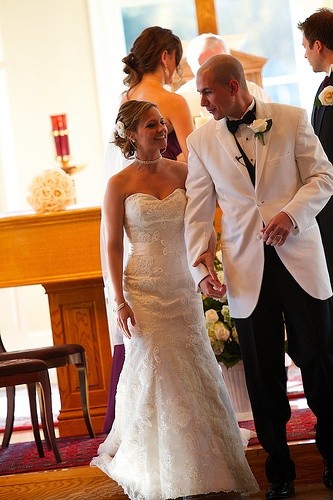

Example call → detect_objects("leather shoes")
323 463 333 489
265 482 296 500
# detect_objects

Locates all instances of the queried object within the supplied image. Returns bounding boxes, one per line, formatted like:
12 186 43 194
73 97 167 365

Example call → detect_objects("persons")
176 34 272 125
184 56 333 500
297 7 333 291
119 26 195 164
89 99 260 499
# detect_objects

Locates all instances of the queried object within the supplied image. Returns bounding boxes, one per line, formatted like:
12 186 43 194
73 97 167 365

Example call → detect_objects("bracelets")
113 301 129 312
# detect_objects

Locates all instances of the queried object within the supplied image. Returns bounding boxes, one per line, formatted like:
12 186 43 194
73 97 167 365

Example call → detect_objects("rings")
277 235 283 239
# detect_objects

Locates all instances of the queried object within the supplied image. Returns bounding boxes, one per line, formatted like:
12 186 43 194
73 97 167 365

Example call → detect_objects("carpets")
0 408 318 475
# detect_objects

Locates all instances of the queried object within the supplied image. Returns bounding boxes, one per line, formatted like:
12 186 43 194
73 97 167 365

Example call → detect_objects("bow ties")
226 103 256 134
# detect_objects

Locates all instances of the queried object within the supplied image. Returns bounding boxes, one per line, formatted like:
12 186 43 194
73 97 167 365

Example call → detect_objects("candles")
51 115 73 165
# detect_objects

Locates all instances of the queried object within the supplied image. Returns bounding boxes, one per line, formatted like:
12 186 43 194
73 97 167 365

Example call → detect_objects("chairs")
0 332 95 451
0 358 63 464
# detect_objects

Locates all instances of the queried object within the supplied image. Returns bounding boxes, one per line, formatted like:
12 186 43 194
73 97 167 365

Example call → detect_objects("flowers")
246 118 273 145
199 287 246 369
314 84 333 107
26 167 76 215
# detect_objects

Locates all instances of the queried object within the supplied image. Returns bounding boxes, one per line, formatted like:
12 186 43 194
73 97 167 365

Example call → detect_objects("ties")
317 76 330 100
